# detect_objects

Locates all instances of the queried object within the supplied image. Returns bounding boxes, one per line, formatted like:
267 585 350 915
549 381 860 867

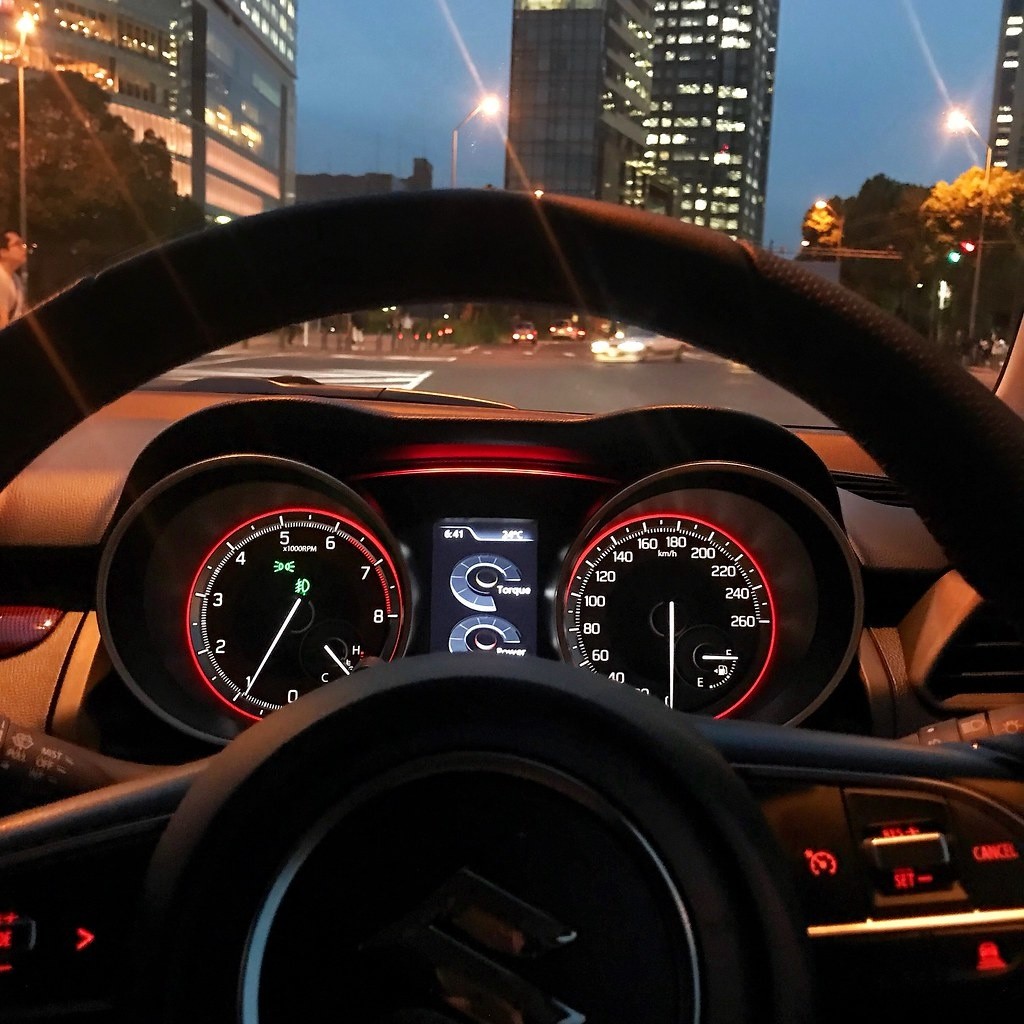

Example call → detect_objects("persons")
1 228 29 330
992 327 1010 370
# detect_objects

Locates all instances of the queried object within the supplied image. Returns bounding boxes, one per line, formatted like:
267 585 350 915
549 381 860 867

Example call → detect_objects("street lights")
451 93 500 187
14 8 42 238
950 110 992 338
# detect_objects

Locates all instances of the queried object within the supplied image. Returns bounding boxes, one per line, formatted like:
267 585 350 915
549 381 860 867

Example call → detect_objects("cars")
591 328 689 364
511 320 538 344
550 319 587 341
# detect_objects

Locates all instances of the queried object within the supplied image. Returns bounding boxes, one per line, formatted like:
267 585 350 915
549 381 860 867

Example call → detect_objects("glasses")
8 240 24 248
